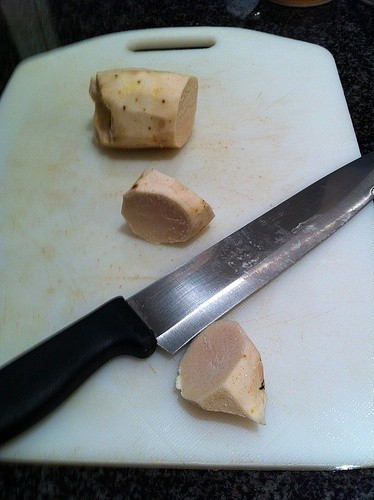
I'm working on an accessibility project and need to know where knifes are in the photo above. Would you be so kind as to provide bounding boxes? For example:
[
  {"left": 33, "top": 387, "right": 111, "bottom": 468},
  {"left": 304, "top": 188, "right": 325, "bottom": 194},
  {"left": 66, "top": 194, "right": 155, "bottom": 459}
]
[{"left": 0, "top": 155, "right": 374, "bottom": 446}]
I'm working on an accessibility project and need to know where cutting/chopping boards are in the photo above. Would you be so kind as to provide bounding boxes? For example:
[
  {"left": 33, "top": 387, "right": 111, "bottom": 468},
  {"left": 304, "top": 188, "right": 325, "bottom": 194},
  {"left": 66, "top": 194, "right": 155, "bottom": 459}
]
[{"left": 0, "top": 26, "right": 374, "bottom": 467}]
